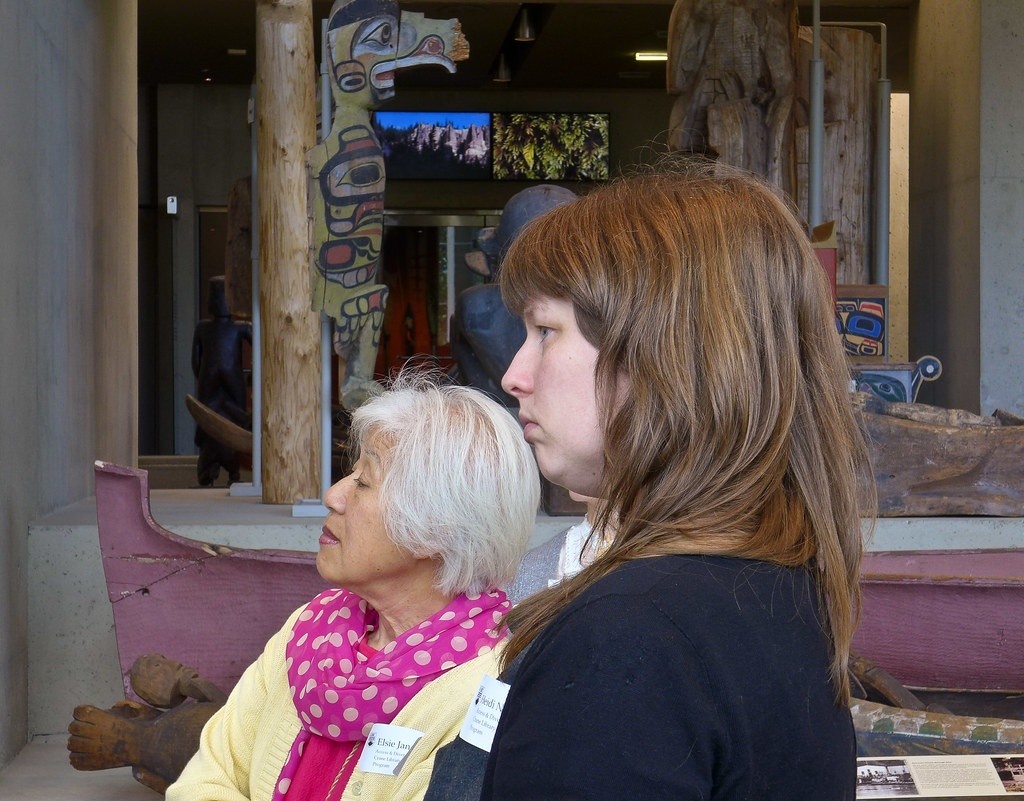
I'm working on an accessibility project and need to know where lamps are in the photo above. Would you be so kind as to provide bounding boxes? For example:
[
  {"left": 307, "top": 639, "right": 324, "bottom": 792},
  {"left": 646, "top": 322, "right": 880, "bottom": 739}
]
[
  {"left": 516, "top": 7, "right": 538, "bottom": 41},
  {"left": 494, "top": 52, "right": 510, "bottom": 81}
]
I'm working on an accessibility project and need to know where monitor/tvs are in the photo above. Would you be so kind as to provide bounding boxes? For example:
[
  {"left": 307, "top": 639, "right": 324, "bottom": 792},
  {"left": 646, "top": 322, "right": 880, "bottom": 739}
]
[{"left": 371, "top": 109, "right": 614, "bottom": 182}]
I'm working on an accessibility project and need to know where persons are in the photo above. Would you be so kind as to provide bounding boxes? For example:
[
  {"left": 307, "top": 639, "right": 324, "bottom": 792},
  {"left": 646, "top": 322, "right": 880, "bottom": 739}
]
[
  {"left": 162, "top": 384, "right": 543, "bottom": 801},
  {"left": 191, "top": 276, "right": 252, "bottom": 485},
  {"left": 450, "top": 186, "right": 580, "bottom": 408},
  {"left": 502, "top": 487, "right": 620, "bottom": 611},
  {"left": 421, "top": 168, "right": 882, "bottom": 801}
]
